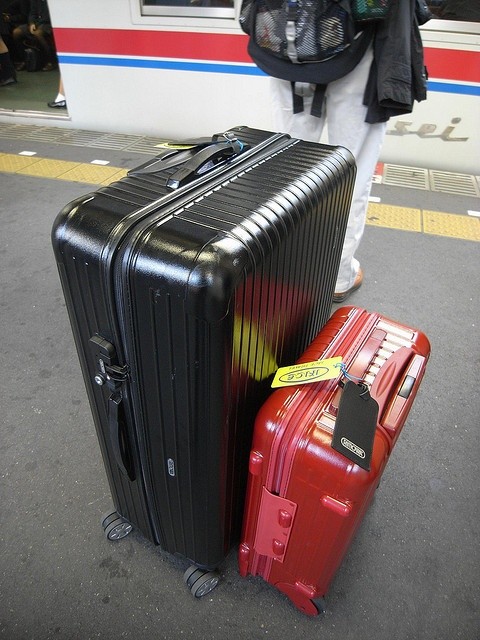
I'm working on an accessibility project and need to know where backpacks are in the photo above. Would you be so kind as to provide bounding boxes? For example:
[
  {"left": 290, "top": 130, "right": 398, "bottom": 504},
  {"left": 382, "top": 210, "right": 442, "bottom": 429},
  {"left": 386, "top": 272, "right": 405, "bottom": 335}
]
[{"left": 240, "top": 0, "right": 387, "bottom": 115}]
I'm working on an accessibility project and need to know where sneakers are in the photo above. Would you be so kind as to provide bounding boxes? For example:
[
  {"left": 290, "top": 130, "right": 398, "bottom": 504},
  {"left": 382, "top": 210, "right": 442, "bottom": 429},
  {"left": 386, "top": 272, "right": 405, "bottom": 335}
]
[{"left": 334, "top": 262, "right": 364, "bottom": 303}]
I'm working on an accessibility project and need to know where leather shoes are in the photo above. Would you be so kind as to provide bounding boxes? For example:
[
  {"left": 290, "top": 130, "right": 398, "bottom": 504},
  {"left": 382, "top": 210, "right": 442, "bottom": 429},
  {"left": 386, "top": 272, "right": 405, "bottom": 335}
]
[{"left": 48, "top": 100, "right": 66, "bottom": 109}]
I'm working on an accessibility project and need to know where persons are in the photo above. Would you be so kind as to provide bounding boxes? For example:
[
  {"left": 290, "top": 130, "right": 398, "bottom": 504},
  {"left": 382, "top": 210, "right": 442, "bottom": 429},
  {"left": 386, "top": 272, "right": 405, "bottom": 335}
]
[
  {"left": 48, "top": 76, "right": 67, "bottom": 108},
  {"left": 266, "top": 0, "right": 389, "bottom": 303},
  {"left": 12, "top": 0, "right": 56, "bottom": 71}
]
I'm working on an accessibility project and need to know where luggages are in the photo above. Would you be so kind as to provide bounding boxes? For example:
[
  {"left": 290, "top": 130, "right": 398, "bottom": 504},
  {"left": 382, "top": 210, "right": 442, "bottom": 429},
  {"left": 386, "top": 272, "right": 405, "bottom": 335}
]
[
  {"left": 237, "top": 307, "right": 430, "bottom": 613},
  {"left": 50, "top": 126, "right": 375, "bottom": 596}
]
